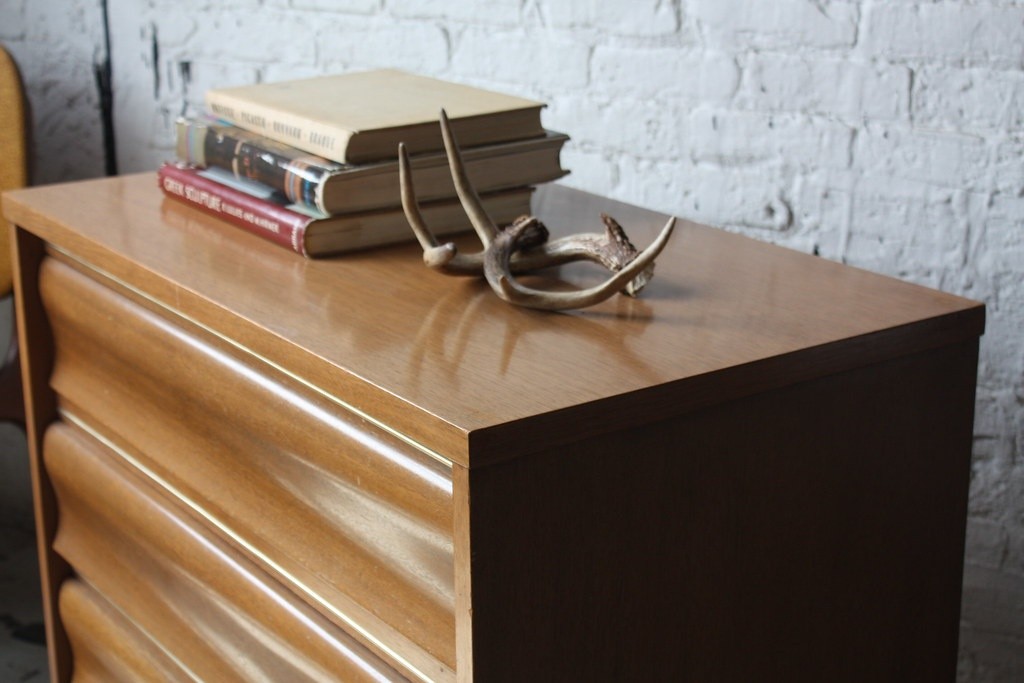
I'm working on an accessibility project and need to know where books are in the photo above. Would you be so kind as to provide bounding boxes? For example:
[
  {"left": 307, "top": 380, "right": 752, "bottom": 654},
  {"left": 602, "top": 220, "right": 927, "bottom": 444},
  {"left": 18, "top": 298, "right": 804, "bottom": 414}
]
[
  {"left": 205, "top": 65, "right": 546, "bottom": 165},
  {"left": 176, "top": 116, "right": 573, "bottom": 215},
  {"left": 157, "top": 162, "right": 538, "bottom": 260}
]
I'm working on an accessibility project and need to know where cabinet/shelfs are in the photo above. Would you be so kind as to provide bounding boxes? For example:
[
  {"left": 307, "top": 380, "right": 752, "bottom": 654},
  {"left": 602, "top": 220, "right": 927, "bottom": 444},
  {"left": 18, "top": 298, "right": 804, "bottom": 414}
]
[{"left": 0, "top": 168, "right": 986, "bottom": 683}]
